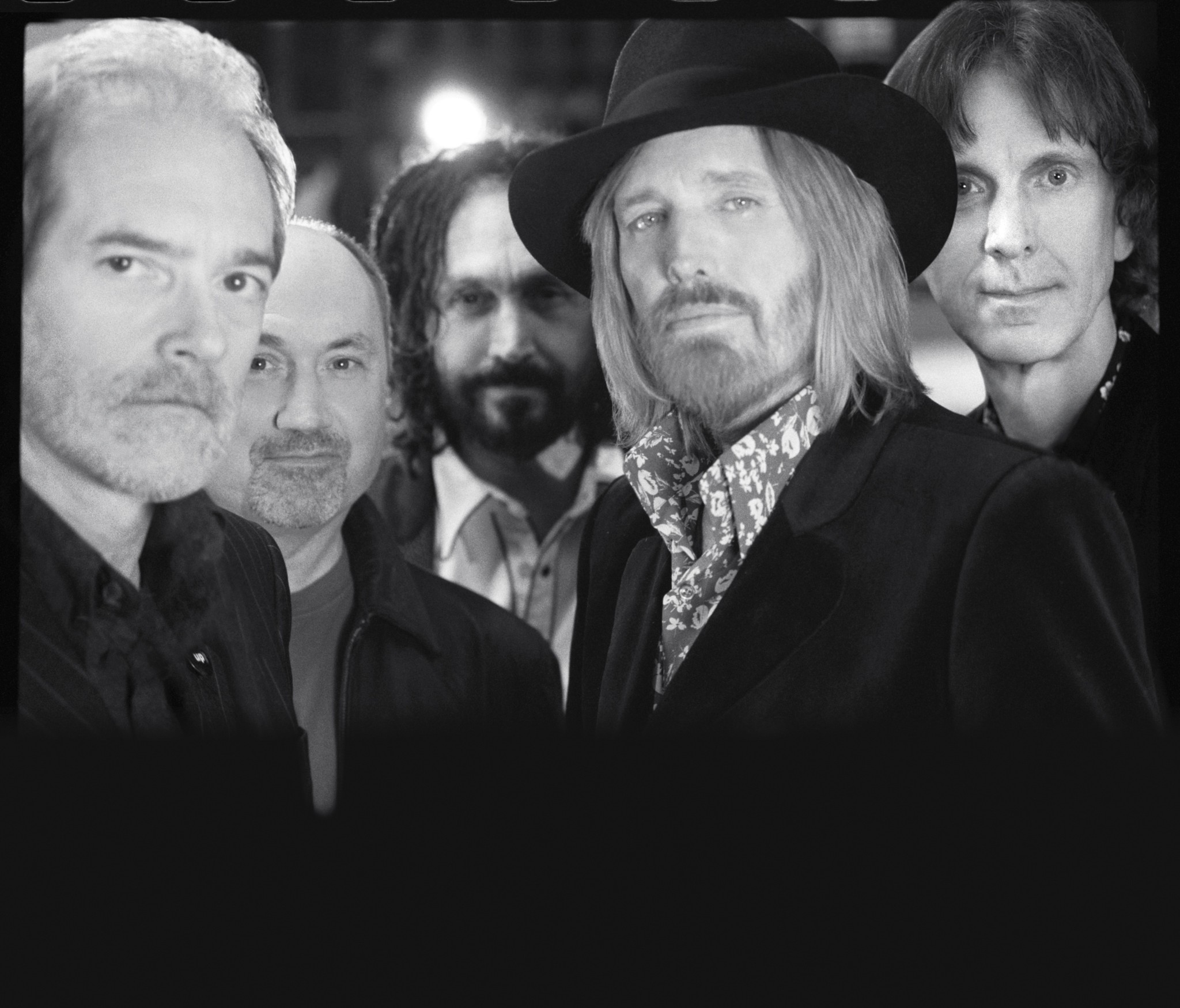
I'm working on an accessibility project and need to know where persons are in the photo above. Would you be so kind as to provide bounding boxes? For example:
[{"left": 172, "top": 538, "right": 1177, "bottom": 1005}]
[
  {"left": 878, "top": 0, "right": 1160, "bottom": 745},
  {"left": 203, "top": 219, "right": 575, "bottom": 1008},
  {"left": 21, "top": 19, "right": 341, "bottom": 1008},
  {"left": 508, "top": 18, "right": 1159, "bottom": 1008},
  {"left": 366, "top": 133, "right": 659, "bottom": 726}
]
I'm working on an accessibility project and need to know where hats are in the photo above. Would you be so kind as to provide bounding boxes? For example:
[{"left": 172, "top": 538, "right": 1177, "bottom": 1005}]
[{"left": 509, "top": 18, "right": 959, "bottom": 296}]
